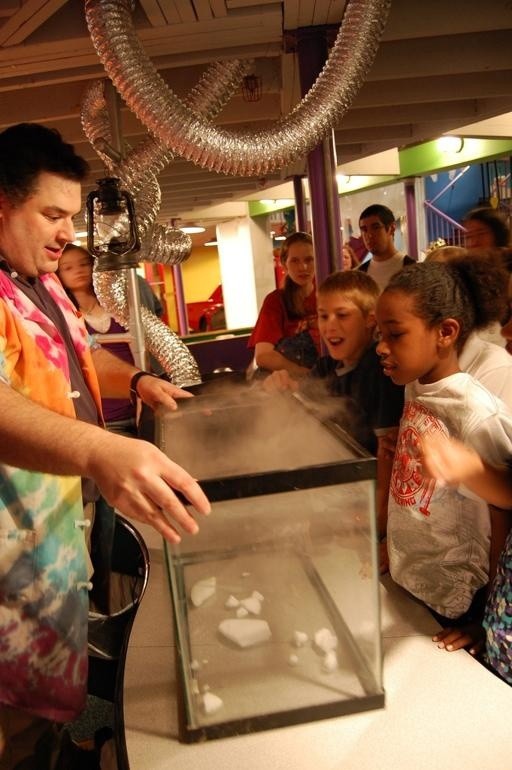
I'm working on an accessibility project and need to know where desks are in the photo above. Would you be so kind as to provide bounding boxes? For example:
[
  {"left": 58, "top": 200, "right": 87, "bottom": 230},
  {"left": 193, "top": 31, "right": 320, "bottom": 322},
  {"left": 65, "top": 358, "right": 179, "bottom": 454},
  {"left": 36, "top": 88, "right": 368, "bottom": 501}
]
[{"left": 114, "top": 504, "right": 512, "bottom": 765}]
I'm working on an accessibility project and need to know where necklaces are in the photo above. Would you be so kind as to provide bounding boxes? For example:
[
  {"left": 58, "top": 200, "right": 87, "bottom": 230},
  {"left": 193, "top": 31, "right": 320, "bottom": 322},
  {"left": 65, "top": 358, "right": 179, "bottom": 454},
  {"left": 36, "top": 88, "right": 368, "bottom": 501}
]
[{"left": 78, "top": 300, "right": 98, "bottom": 315}]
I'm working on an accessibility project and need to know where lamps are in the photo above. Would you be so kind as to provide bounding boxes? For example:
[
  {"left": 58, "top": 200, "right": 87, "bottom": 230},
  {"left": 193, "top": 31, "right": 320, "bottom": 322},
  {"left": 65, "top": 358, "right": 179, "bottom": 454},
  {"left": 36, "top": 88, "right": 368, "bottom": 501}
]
[{"left": 86, "top": 176, "right": 142, "bottom": 273}]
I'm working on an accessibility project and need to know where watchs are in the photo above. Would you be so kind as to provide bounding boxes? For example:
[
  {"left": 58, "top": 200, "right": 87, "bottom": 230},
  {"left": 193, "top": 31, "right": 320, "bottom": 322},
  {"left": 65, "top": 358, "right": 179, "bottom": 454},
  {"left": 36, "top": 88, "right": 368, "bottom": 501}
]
[{"left": 377, "top": 536, "right": 388, "bottom": 545}]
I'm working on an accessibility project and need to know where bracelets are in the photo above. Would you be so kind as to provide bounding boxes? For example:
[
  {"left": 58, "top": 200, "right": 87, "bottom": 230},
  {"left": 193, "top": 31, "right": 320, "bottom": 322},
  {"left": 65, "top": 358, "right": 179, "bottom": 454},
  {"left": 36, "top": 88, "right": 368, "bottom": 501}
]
[{"left": 129, "top": 370, "right": 160, "bottom": 406}]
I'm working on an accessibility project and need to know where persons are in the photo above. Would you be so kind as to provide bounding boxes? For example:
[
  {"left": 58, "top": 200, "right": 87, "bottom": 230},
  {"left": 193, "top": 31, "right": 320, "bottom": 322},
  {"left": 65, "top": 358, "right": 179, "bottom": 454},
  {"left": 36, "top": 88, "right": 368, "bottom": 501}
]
[
  {"left": 135, "top": 274, "right": 162, "bottom": 372},
  {"left": 0, "top": 122, "right": 211, "bottom": 769},
  {"left": 374, "top": 252, "right": 512, "bottom": 623},
  {"left": 343, "top": 204, "right": 418, "bottom": 291},
  {"left": 245, "top": 232, "right": 322, "bottom": 379},
  {"left": 55, "top": 243, "right": 156, "bottom": 440},
  {"left": 465, "top": 206, "right": 512, "bottom": 270},
  {"left": 341, "top": 244, "right": 361, "bottom": 271},
  {"left": 424, "top": 246, "right": 511, "bottom": 684},
  {"left": 312, "top": 270, "right": 404, "bottom": 576}
]
